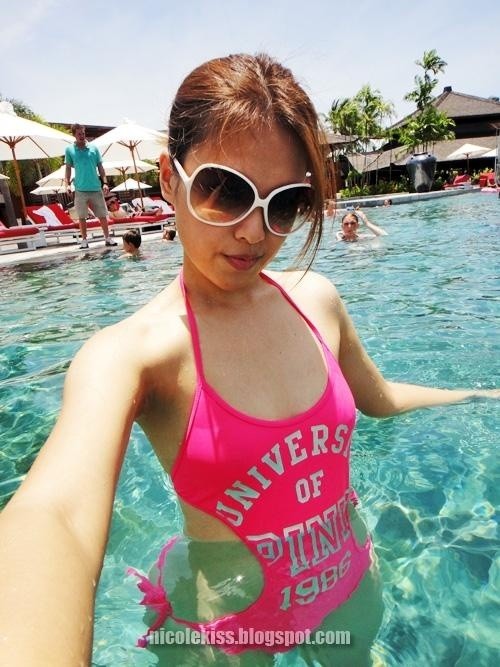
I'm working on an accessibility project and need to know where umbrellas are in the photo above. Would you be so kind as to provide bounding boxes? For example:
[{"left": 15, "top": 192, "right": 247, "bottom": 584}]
[
  {"left": 1, "top": 113, "right": 171, "bottom": 226},
  {"left": 447, "top": 143, "right": 492, "bottom": 175},
  {"left": 483, "top": 148, "right": 499, "bottom": 157}
]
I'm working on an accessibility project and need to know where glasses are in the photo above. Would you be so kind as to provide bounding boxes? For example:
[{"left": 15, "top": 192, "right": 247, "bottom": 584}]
[{"left": 173, "top": 156, "right": 317, "bottom": 238}]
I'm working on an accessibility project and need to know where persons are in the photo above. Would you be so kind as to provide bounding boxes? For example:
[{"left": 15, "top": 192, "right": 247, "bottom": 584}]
[
  {"left": 65, "top": 122, "right": 119, "bottom": 249},
  {"left": 383, "top": 198, "right": 393, "bottom": 208},
  {"left": 155, "top": 228, "right": 177, "bottom": 243},
  {"left": 325, "top": 200, "right": 339, "bottom": 216},
  {"left": 0, "top": 47, "right": 500, "bottom": 666},
  {"left": 336, "top": 209, "right": 388, "bottom": 243},
  {"left": 116, "top": 229, "right": 143, "bottom": 259}
]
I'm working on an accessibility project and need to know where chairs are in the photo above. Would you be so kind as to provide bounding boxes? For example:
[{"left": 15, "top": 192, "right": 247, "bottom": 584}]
[{"left": 0, "top": 195, "right": 176, "bottom": 255}]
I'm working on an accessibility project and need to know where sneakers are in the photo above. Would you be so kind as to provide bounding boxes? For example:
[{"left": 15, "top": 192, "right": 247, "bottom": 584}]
[
  {"left": 105, "top": 238, "right": 118, "bottom": 246},
  {"left": 80, "top": 239, "right": 89, "bottom": 249}
]
